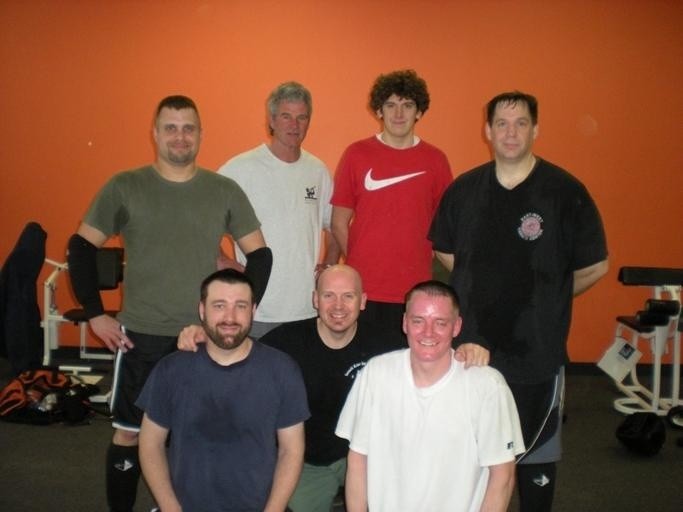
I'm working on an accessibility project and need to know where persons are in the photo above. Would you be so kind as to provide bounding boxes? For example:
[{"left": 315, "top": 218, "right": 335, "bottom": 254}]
[
  {"left": 134, "top": 270, "right": 313, "bottom": 512},
  {"left": 334, "top": 280, "right": 526, "bottom": 512},
  {"left": 427, "top": 90, "right": 611, "bottom": 512},
  {"left": 65, "top": 94, "right": 273, "bottom": 511},
  {"left": 177, "top": 264, "right": 492, "bottom": 512},
  {"left": 328, "top": 67, "right": 454, "bottom": 357},
  {"left": 215, "top": 81, "right": 336, "bottom": 341}
]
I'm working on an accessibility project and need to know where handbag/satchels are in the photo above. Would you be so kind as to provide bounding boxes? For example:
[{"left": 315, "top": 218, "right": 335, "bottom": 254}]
[{"left": 0, "top": 365, "right": 100, "bottom": 425}]
